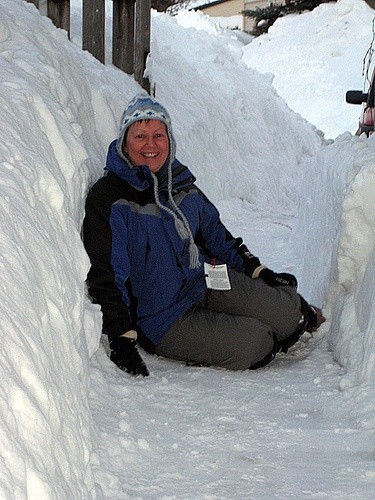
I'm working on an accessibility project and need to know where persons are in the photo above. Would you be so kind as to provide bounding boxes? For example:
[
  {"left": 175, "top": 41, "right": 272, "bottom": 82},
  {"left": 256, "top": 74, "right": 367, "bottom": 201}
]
[{"left": 80, "top": 92, "right": 325, "bottom": 379}]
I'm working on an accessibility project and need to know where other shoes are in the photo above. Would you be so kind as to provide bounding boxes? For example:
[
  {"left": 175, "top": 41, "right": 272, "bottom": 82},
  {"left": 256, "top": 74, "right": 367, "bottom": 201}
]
[{"left": 305, "top": 303, "right": 326, "bottom": 333}]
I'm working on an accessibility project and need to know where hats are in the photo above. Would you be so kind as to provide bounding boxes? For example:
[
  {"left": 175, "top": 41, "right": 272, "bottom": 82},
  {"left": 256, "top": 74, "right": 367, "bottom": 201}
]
[{"left": 117, "top": 92, "right": 202, "bottom": 269}]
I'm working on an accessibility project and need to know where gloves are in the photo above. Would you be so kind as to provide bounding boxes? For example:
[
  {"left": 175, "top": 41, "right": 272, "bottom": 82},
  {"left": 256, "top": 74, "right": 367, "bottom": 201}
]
[
  {"left": 259, "top": 267, "right": 297, "bottom": 288},
  {"left": 110, "top": 337, "right": 149, "bottom": 376}
]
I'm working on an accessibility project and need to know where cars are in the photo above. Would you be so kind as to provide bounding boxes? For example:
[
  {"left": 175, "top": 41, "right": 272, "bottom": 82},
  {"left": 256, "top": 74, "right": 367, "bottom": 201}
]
[{"left": 346, "top": 67, "right": 375, "bottom": 137}]
